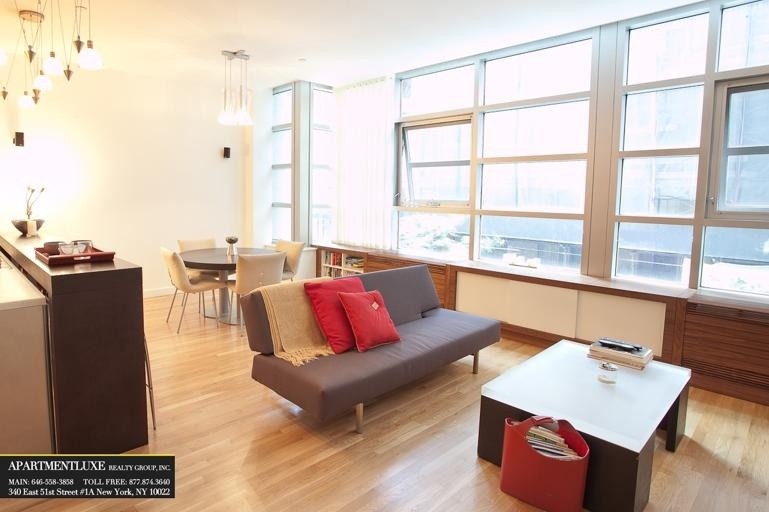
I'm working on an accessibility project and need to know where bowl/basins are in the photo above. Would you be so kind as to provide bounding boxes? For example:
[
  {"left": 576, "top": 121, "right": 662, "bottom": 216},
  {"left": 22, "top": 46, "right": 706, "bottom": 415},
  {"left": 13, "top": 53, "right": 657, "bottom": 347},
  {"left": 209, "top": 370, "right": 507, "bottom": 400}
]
[{"left": 44, "top": 242, "right": 64, "bottom": 255}]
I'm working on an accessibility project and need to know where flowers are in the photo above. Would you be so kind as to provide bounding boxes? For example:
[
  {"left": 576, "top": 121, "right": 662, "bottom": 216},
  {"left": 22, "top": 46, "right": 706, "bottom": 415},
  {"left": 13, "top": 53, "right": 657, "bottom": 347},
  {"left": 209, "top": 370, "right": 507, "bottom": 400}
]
[{"left": 24, "top": 185, "right": 46, "bottom": 219}]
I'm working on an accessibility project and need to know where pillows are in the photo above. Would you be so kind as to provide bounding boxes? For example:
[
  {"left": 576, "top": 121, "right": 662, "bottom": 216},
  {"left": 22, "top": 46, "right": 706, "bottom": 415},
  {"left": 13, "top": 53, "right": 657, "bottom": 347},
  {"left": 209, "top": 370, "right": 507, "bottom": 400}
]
[
  {"left": 337, "top": 289, "right": 401, "bottom": 353},
  {"left": 303, "top": 277, "right": 366, "bottom": 355}
]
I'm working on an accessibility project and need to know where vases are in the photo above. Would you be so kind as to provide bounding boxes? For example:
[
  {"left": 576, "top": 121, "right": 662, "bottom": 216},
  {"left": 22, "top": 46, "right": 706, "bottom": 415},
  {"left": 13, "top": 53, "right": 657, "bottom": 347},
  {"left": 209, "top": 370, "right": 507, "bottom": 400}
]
[{"left": 11, "top": 219, "right": 45, "bottom": 236}]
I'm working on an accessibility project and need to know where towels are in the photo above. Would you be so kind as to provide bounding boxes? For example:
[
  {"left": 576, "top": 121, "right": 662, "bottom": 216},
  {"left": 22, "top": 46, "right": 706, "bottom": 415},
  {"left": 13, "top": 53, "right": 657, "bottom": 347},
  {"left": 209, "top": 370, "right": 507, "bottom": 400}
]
[{"left": 26, "top": 220, "right": 38, "bottom": 238}]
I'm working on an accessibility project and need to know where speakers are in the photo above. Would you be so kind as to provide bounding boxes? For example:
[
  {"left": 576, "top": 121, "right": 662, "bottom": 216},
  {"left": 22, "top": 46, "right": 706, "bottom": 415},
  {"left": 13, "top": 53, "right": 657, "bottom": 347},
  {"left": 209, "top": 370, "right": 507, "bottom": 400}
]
[
  {"left": 14, "top": 131, "right": 25, "bottom": 147},
  {"left": 223, "top": 146, "right": 231, "bottom": 157}
]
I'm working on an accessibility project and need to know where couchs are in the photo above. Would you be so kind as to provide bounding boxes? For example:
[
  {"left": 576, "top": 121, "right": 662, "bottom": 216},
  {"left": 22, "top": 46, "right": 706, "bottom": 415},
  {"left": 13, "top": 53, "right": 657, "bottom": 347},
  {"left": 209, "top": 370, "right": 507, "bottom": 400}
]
[{"left": 238, "top": 264, "right": 500, "bottom": 434}]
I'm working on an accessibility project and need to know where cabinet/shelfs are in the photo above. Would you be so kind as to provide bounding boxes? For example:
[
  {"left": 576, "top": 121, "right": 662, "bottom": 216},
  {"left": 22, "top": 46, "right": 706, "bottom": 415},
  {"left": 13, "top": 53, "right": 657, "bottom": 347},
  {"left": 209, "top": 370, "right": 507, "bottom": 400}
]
[{"left": 320, "top": 249, "right": 365, "bottom": 279}]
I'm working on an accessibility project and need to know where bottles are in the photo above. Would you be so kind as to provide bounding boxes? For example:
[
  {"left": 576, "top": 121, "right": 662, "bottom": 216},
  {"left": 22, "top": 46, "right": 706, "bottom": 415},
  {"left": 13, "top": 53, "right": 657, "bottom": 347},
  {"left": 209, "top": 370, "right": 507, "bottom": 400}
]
[{"left": 598, "top": 362, "right": 618, "bottom": 384}]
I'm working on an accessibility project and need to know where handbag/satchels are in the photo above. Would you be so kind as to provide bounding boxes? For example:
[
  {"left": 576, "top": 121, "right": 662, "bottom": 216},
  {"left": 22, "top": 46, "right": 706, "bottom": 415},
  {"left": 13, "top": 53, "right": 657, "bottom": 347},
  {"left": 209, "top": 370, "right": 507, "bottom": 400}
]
[{"left": 500, "top": 414, "right": 590, "bottom": 512}]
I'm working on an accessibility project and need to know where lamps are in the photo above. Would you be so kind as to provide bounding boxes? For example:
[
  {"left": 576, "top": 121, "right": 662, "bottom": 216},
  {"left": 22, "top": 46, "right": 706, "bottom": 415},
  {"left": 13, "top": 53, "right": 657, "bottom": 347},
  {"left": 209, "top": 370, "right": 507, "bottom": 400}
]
[
  {"left": 219, "top": 50, "right": 254, "bottom": 127},
  {"left": 2, "top": 0, "right": 102, "bottom": 112}
]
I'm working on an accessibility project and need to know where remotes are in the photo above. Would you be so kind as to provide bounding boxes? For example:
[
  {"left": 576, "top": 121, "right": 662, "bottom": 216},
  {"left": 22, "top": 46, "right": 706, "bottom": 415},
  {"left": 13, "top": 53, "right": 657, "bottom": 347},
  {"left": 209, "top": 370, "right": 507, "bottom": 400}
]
[
  {"left": 598, "top": 340, "right": 634, "bottom": 351},
  {"left": 602, "top": 338, "right": 642, "bottom": 351}
]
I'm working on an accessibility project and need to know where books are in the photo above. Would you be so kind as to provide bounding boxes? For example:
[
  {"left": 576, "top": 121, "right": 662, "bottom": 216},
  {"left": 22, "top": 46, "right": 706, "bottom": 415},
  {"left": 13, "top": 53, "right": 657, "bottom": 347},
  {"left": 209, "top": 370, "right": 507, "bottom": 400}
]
[
  {"left": 325, "top": 251, "right": 365, "bottom": 278},
  {"left": 510, "top": 420, "right": 583, "bottom": 460},
  {"left": 587, "top": 337, "right": 654, "bottom": 371}
]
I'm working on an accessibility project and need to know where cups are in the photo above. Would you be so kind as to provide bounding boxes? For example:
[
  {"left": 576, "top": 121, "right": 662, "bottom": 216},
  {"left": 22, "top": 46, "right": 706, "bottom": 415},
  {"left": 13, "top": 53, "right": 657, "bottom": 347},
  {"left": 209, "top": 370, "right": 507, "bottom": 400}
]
[
  {"left": 511, "top": 253, "right": 540, "bottom": 267},
  {"left": 59, "top": 240, "right": 93, "bottom": 255},
  {"left": 27, "top": 218, "right": 37, "bottom": 237}
]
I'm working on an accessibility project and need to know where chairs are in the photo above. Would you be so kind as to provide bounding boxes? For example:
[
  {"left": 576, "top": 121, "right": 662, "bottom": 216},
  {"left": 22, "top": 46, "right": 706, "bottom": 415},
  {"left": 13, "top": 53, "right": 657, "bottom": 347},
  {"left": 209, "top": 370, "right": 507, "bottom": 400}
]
[
  {"left": 179, "top": 239, "right": 216, "bottom": 307},
  {"left": 226, "top": 252, "right": 287, "bottom": 337},
  {"left": 160, "top": 247, "right": 228, "bottom": 334},
  {"left": 275, "top": 238, "right": 304, "bottom": 282}
]
